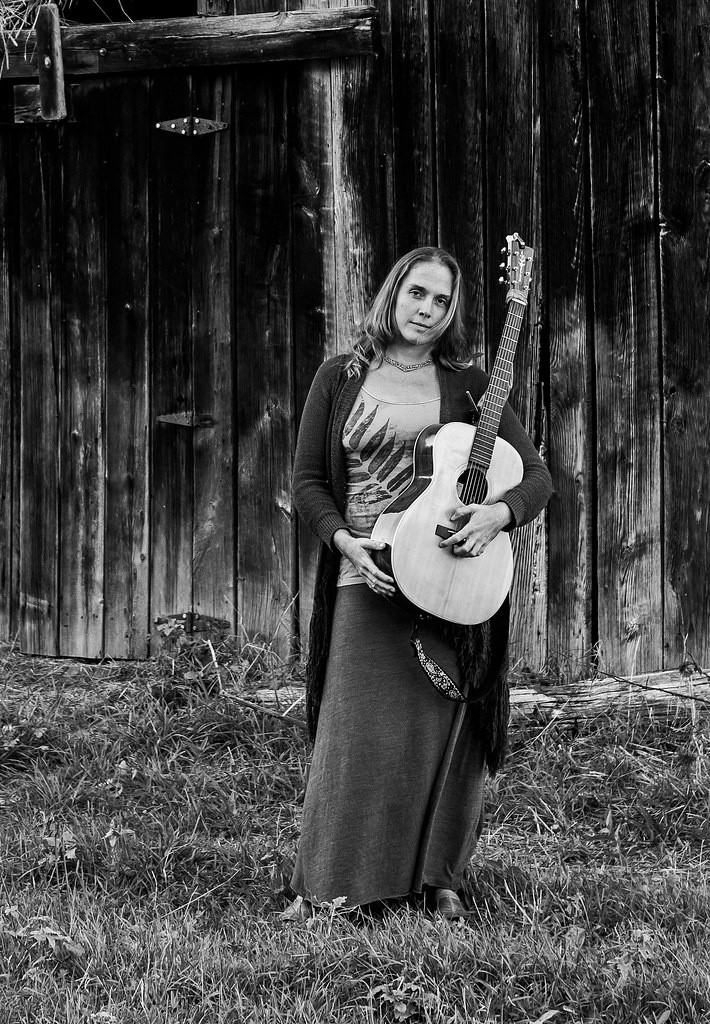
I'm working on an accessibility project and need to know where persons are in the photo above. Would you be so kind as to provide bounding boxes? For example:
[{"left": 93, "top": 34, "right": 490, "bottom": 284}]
[{"left": 275, "top": 245, "right": 552, "bottom": 922}]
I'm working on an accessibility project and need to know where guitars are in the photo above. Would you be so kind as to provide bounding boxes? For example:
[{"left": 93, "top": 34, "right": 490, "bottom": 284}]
[{"left": 370, "top": 232, "right": 535, "bottom": 628}]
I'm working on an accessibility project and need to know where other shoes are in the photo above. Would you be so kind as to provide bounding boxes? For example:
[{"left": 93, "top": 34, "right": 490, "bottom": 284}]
[
  {"left": 434, "top": 890, "right": 468, "bottom": 921},
  {"left": 278, "top": 898, "right": 312, "bottom": 922}
]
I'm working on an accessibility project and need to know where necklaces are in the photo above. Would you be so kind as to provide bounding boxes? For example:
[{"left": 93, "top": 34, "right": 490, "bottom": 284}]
[{"left": 382, "top": 351, "right": 434, "bottom": 372}]
